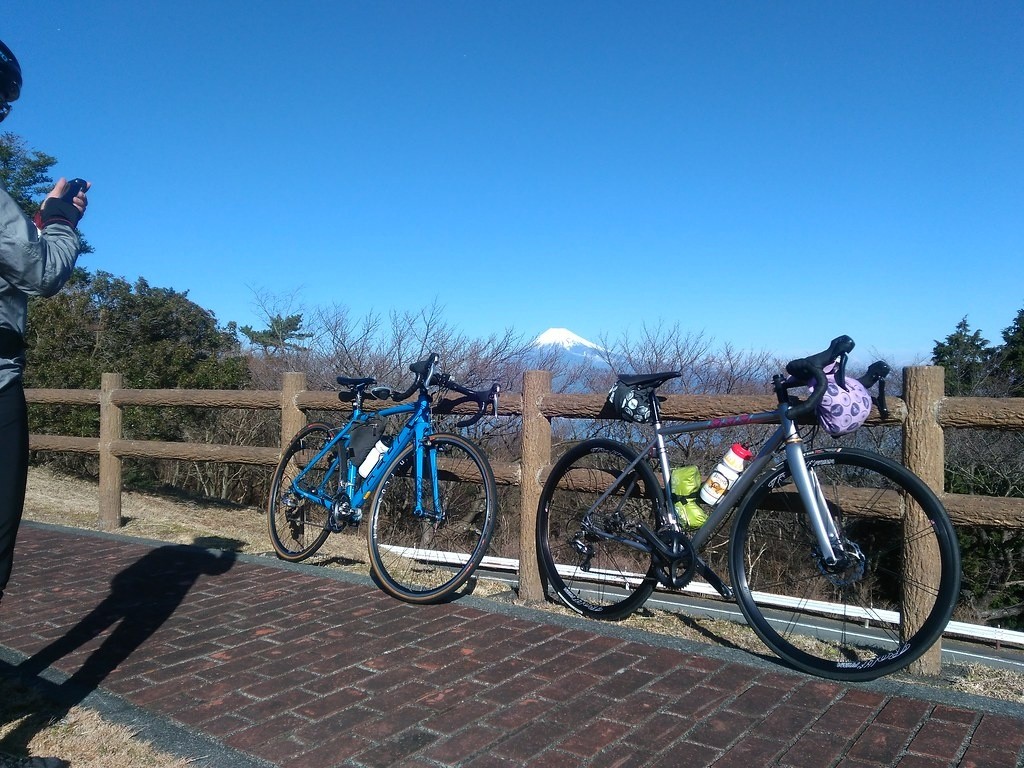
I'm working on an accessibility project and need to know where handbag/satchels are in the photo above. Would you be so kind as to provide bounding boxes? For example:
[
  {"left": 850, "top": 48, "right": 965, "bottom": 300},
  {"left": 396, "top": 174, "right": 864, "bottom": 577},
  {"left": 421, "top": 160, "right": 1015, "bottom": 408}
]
[{"left": 349, "top": 412, "right": 388, "bottom": 466}]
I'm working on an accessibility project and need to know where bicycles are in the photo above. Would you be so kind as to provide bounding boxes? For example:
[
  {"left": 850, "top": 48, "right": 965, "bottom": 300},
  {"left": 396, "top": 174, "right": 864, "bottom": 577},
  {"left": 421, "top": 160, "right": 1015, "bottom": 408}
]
[
  {"left": 534, "top": 334, "right": 964, "bottom": 683},
  {"left": 264, "top": 351, "right": 503, "bottom": 606}
]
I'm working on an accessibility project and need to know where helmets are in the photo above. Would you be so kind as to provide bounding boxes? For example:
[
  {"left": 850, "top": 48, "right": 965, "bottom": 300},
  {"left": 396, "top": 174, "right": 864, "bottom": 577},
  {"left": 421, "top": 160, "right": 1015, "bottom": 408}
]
[{"left": 0, "top": 40, "right": 22, "bottom": 122}]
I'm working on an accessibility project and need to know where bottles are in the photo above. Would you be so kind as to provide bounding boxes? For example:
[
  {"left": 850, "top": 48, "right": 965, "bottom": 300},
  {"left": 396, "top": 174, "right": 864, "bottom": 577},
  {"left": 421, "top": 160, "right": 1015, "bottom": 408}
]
[
  {"left": 700, "top": 442, "right": 752, "bottom": 507},
  {"left": 358, "top": 436, "right": 393, "bottom": 479}
]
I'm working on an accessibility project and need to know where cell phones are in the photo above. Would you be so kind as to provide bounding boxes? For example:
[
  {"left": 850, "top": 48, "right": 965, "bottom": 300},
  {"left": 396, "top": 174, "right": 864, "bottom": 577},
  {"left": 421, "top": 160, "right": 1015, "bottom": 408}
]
[{"left": 60, "top": 177, "right": 87, "bottom": 205}]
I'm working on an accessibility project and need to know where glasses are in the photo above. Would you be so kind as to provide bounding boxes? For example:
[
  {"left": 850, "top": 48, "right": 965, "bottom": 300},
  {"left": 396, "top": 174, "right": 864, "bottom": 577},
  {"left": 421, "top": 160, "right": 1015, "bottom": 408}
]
[{"left": 0, "top": 93, "right": 12, "bottom": 122}]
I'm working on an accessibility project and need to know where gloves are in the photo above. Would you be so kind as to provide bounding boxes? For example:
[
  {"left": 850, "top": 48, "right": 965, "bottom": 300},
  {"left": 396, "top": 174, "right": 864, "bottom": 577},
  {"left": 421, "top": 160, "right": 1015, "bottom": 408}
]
[{"left": 34, "top": 198, "right": 80, "bottom": 231}]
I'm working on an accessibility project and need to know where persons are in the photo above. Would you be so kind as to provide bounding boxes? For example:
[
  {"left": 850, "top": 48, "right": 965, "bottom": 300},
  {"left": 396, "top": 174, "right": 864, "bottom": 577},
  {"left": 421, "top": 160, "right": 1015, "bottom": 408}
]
[{"left": 0, "top": 41, "right": 90, "bottom": 600}]
